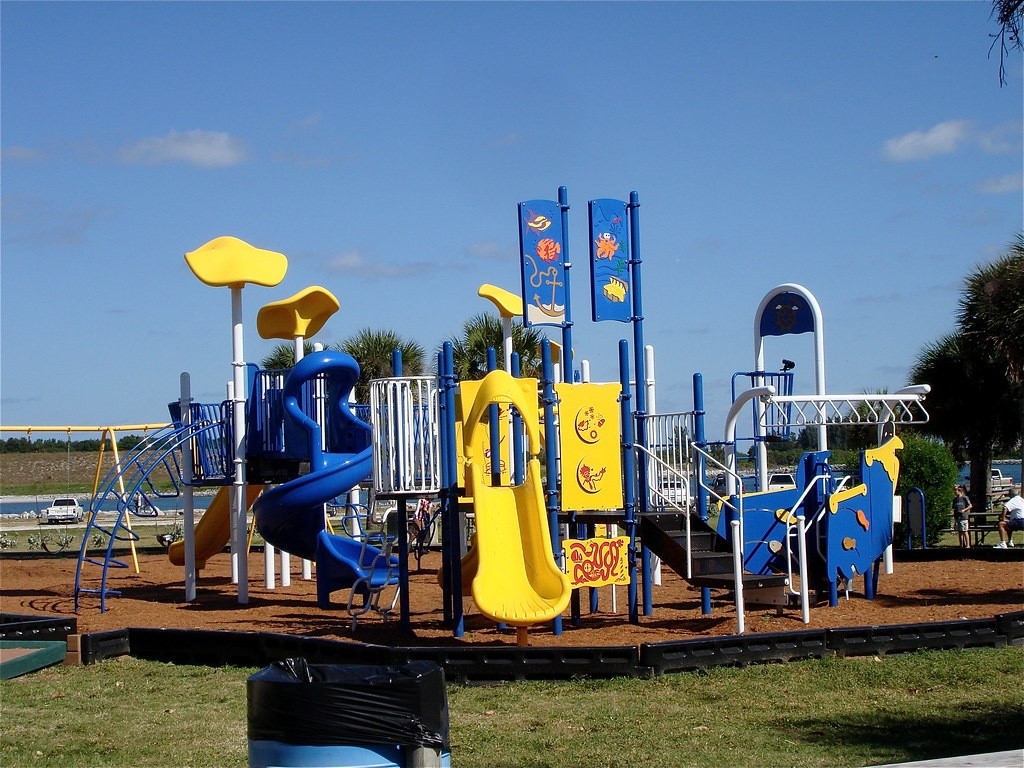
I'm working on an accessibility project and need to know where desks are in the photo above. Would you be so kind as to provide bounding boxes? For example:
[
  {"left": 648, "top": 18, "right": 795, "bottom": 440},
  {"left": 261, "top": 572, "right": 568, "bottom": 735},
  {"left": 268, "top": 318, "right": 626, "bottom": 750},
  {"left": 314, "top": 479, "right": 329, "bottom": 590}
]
[{"left": 940, "top": 513, "right": 1011, "bottom": 546}]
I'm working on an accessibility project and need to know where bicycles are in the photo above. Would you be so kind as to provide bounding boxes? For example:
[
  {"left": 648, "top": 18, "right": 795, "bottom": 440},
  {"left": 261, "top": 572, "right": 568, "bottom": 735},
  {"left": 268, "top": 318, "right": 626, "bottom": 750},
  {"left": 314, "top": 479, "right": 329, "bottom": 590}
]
[{"left": 127, "top": 499, "right": 154, "bottom": 515}]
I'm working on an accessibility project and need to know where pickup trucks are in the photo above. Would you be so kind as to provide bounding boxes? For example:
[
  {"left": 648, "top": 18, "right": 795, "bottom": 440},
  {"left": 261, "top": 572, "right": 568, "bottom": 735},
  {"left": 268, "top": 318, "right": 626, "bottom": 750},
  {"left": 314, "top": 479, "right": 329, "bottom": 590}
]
[
  {"left": 964, "top": 468, "right": 1013, "bottom": 492},
  {"left": 46, "top": 497, "right": 84, "bottom": 524}
]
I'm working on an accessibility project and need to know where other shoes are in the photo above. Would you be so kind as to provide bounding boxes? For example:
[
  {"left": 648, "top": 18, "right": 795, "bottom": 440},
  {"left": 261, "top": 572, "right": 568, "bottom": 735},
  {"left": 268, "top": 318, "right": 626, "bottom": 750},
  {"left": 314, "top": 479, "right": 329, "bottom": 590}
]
[{"left": 966, "top": 546, "right": 970, "bottom": 548}]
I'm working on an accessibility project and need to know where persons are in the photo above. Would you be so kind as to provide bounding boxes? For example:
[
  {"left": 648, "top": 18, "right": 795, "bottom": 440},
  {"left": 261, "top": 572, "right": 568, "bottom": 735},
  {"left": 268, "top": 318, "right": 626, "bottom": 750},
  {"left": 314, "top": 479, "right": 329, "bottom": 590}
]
[
  {"left": 993, "top": 488, "right": 1024, "bottom": 549},
  {"left": 951, "top": 485, "right": 973, "bottom": 549}
]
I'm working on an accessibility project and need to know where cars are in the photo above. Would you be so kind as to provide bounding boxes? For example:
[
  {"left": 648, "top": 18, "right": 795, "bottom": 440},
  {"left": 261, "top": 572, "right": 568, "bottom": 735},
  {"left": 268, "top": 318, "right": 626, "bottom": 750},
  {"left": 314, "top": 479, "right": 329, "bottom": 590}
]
[
  {"left": 767, "top": 472, "right": 797, "bottom": 491},
  {"left": 658, "top": 480, "right": 686, "bottom": 505},
  {"left": 833, "top": 476, "right": 848, "bottom": 491},
  {"left": 708, "top": 472, "right": 746, "bottom": 504}
]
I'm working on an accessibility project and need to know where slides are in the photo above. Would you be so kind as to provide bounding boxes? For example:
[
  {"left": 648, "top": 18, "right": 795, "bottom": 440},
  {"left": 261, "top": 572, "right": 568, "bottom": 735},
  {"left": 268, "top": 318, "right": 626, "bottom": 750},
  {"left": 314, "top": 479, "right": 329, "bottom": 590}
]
[
  {"left": 438, "top": 369, "right": 573, "bottom": 627},
  {"left": 251, "top": 350, "right": 410, "bottom": 602},
  {"left": 168, "top": 440, "right": 262, "bottom": 567}
]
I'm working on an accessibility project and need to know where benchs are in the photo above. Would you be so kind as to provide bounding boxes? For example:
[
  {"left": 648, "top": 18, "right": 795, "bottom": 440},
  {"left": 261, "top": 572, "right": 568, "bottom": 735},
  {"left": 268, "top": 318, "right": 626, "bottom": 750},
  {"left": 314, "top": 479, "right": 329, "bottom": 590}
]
[
  {"left": 941, "top": 529, "right": 984, "bottom": 546},
  {"left": 970, "top": 526, "right": 1008, "bottom": 546}
]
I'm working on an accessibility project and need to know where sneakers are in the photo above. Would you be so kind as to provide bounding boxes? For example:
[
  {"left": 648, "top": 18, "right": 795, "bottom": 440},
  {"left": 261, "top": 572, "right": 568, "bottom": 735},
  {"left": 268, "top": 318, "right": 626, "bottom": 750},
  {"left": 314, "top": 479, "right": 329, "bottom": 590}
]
[
  {"left": 993, "top": 543, "right": 1007, "bottom": 549},
  {"left": 1006, "top": 542, "right": 1014, "bottom": 547}
]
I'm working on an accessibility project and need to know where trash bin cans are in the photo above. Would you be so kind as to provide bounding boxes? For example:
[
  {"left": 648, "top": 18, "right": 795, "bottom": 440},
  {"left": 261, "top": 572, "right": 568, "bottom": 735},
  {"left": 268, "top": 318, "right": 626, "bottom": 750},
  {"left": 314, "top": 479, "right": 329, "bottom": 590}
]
[{"left": 246, "top": 663, "right": 454, "bottom": 768}]
